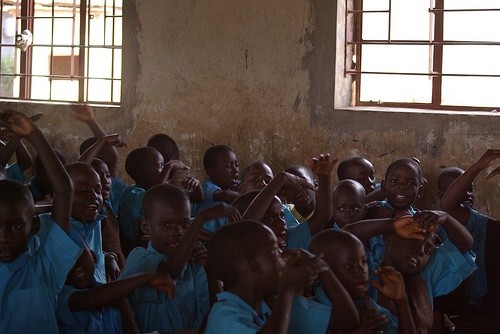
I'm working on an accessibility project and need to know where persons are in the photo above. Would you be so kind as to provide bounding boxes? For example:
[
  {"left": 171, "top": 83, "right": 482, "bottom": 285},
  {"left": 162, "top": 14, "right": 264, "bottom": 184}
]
[
  {"left": 202, "top": 219, "right": 360, "bottom": 334},
  {"left": 0, "top": 102, "right": 500, "bottom": 334}
]
